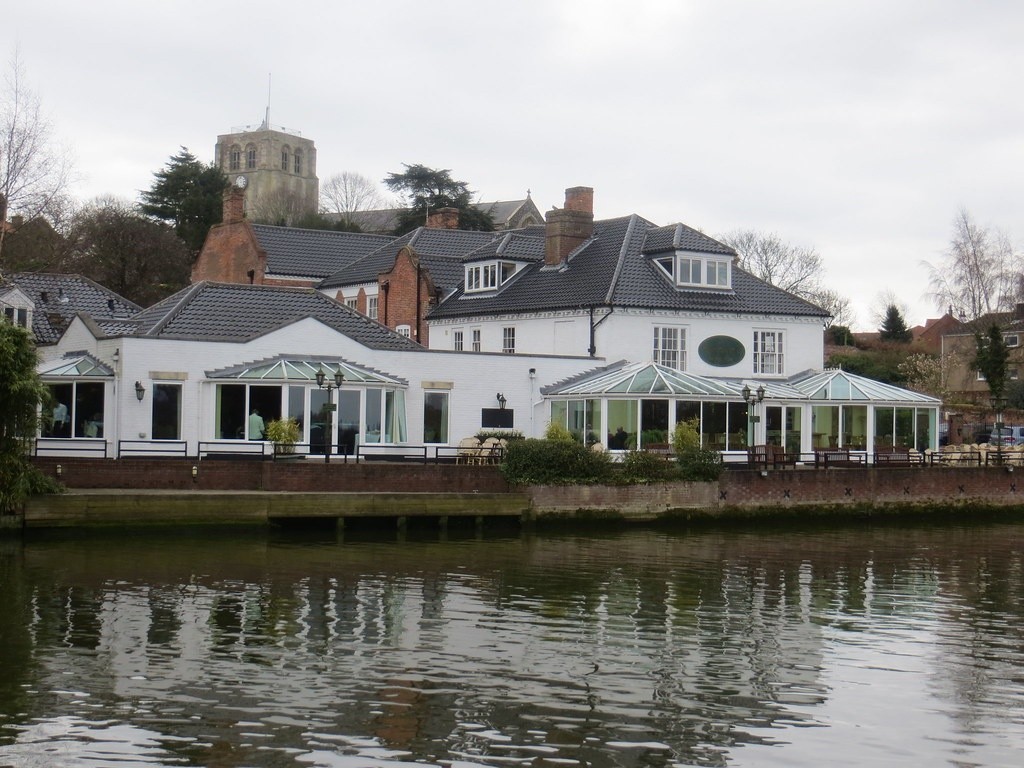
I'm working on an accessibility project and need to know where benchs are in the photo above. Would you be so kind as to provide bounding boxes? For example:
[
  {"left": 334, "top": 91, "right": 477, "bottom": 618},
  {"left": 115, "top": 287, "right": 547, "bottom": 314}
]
[
  {"left": 747, "top": 444, "right": 796, "bottom": 470},
  {"left": 815, "top": 447, "right": 862, "bottom": 467},
  {"left": 876, "top": 446, "right": 922, "bottom": 467}
]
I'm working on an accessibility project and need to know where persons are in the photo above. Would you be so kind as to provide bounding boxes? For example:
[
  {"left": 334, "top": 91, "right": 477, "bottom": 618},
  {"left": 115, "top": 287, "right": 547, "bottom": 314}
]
[
  {"left": 249, "top": 409, "right": 266, "bottom": 441},
  {"left": 51, "top": 398, "right": 67, "bottom": 438}
]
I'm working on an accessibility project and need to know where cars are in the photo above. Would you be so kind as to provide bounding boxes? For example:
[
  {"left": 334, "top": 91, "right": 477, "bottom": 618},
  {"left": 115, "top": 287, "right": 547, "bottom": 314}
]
[{"left": 989, "top": 426, "right": 1024, "bottom": 446}]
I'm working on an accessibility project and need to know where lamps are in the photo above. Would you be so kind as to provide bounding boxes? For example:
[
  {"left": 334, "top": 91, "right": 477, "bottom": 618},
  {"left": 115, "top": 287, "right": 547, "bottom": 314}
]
[
  {"left": 192, "top": 466, "right": 198, "bottom": 484},
  {"left": 135, "top": 381, "right": 145, "bottom": 402},
  {"left": 56, "top": 464, "right": 62, "bottom": 477},
  {"left": 497, "top": 392, "right": 506, "bottom": 412}
]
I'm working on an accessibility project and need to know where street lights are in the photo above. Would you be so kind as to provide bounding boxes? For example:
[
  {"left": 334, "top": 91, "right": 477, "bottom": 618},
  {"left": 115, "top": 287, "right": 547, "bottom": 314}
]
[
  {"left": 742, "top": 384, "right": 765, "bottom": 446},
  {"left": 989, "top": 393, "right": 1009, "bottom": 464}
]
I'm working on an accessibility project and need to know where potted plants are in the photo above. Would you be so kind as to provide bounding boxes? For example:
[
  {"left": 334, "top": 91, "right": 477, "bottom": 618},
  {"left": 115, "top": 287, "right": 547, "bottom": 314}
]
[{"left": 267, "top": 416, "right": 302, "bottom": 462}]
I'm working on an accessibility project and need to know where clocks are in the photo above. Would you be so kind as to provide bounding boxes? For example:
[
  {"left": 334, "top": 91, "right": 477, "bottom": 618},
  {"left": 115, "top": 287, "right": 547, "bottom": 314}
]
[{"left": 235, "top": 175, "right": 248, "bottom": 190}]
